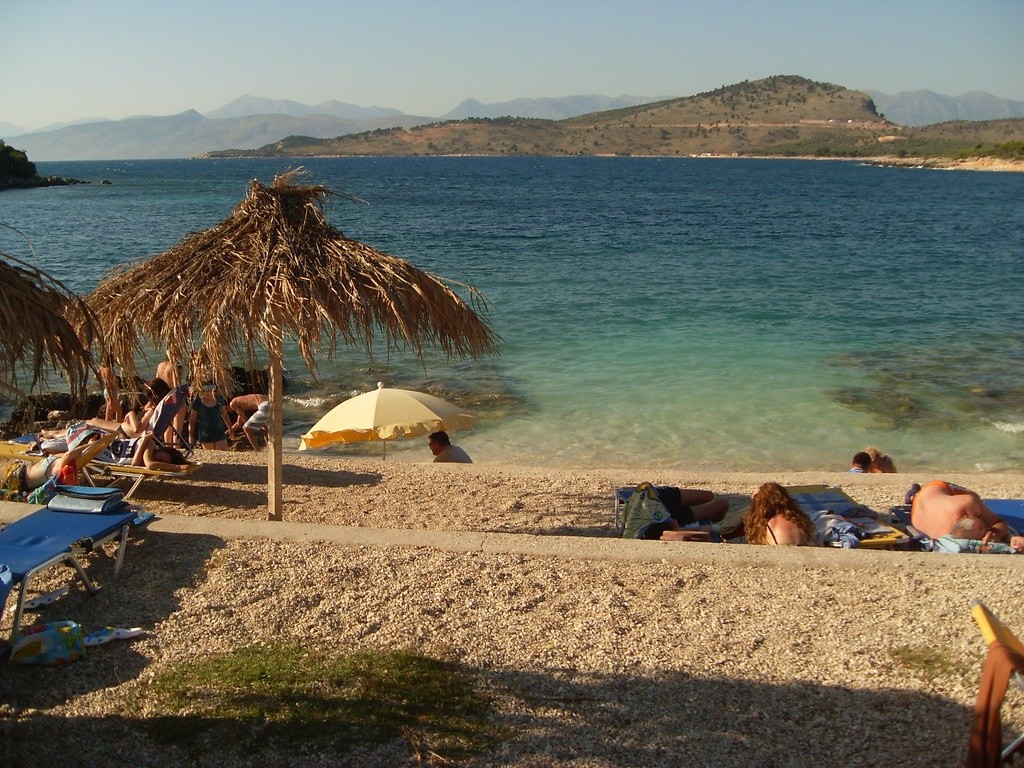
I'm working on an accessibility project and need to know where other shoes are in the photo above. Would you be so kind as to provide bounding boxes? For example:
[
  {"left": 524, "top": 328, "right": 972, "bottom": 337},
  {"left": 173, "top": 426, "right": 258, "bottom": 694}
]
[{"left": 904, "top": 483, "right": 921, "bottom": 504}]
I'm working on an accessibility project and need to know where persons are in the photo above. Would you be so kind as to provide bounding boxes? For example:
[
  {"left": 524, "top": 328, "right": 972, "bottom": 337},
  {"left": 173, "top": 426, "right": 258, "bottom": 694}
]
[
  {"left": 0, "top": 378, "right": 185, "bottom": 492},
  {"left": 155, "top": 348, "right": 185, "bottom": 449},
  {"left": 904, "top": 480, "right": 1024, "bottom": 555},
  {"left": 428, "top": 430, "right": 473, "bottom": 463},
  {"left": 622, "top": 482, "right": 729, "bottom": 539},
  {"left": 848, "top": 447, "right": 897, "bottom": 473},
  {"left": 224, "top": 394, "right": 269, "bottom": 452},
  {"left": 743, "top": 481, "right": 816, "bottom": 546},
  {"left": 188, "top": 381, "right": 234, "bottom": 450},
  {"left": 93, "top": 352, "right": 122, "bottom": 422}
]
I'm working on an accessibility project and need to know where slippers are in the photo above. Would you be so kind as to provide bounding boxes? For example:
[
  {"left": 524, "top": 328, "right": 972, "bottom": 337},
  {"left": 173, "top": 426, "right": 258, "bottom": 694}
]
[
  {"left": 83, "top": 626, "right": 142, "bottom": 647},
  {"left": 23, "top": 587, "right": 68, "bottom": 609}
]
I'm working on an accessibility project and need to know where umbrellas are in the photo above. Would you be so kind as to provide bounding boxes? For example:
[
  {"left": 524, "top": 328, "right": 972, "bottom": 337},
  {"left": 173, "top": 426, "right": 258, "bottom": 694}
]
[
  {"left": 298, "top": 382, "right": 479, "bottom": 461},
  {"left": 0, "top": 250, "right": 125, "bottom": 437},
  {"left": 55, "top": 165, "right": 507, "bottom": 521}
]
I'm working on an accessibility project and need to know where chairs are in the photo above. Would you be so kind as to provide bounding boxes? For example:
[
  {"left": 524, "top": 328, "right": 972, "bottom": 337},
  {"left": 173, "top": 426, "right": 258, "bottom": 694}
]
[
  {"left": 887, "top": 505, "right": 1020, "bottom": 554},
  {"left": 0, "top": 383, "right": 195, "bottom": 645},
  {"left": 614, "top": 485, "right": 724, "bottom": 544},
  {"left": 787, "top": 485, "right": 911, "bottom": 552},
  {"left": 972, "top": 603, "right": 1024, "bottom": 768}
]
